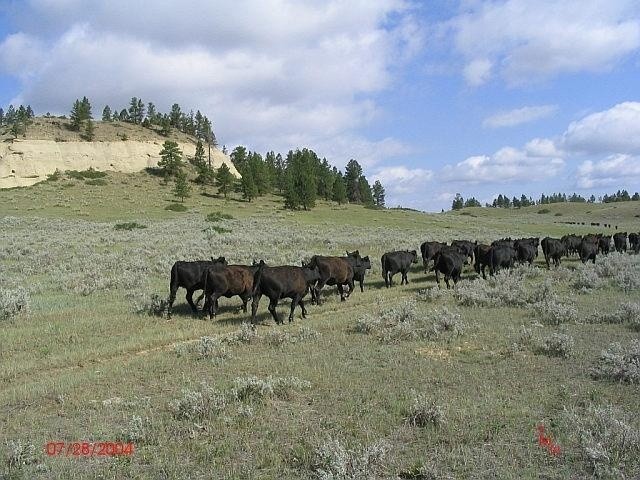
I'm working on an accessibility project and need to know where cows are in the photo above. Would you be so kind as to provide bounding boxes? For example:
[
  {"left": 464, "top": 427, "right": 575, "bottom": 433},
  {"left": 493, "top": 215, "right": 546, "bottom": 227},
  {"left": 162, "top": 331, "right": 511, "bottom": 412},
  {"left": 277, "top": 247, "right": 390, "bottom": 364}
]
[
  {"left": 166, "top": 256, "right": 229, "bottom": 316},
  {"left": 195, "top": 258, "right": 266, "bottom": 320},
  {"left": 381, "top": 249, "right": 418, "bottom": 287},
  {"left": 309, "top": 250, "right": 371, "bottom": 305},
  {"left": 421, "top": 231, "right": 640, "bottom": 288},
  {"left": 248, "top": 260, "right": 321, "bottom": 326}
]
